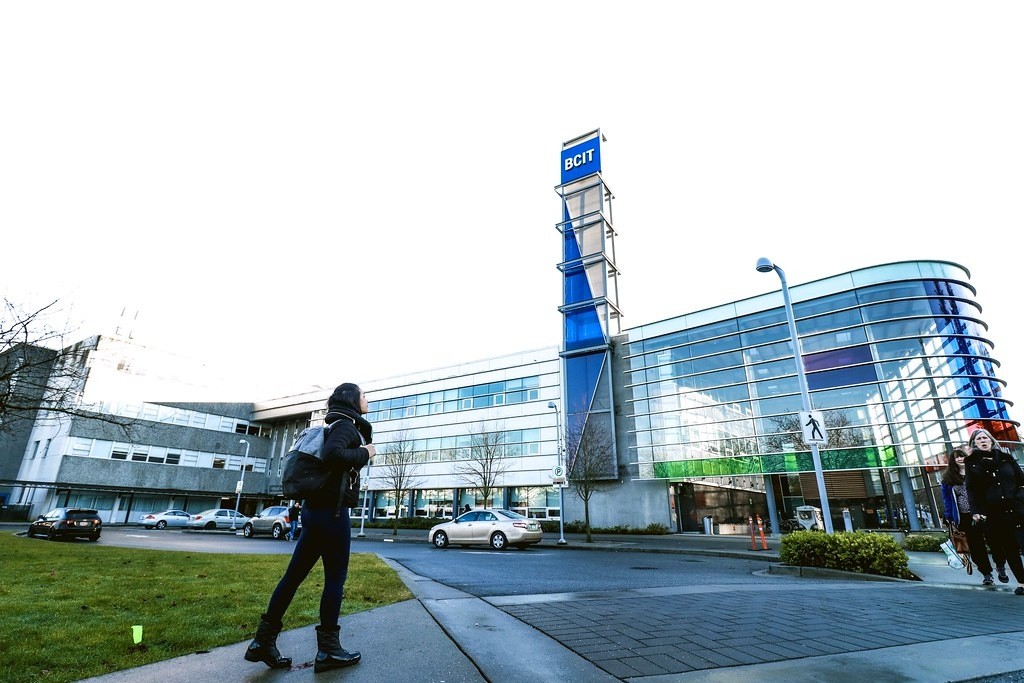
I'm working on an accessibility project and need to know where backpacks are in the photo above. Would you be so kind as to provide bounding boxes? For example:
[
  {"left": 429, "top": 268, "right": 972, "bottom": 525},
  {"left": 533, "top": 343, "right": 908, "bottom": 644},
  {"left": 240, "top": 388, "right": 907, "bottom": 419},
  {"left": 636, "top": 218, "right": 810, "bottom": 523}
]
[{"left": 282, "top": 419, "right": 366, "bottom": 500}]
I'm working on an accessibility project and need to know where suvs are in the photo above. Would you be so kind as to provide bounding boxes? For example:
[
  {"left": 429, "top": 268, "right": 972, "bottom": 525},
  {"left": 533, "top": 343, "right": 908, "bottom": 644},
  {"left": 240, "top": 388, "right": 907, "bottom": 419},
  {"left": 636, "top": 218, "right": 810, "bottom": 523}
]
[{"left": 242, "top": 506, "right": 303, "bottom": 540}]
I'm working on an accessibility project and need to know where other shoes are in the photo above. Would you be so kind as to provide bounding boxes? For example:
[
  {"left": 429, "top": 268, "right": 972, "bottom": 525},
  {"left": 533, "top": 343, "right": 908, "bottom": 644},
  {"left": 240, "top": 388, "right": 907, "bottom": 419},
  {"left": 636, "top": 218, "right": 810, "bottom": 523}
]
[
  {"left": 289, "top": 538, "right": 293, "bottom": 542},
  {"left": 995, "top": 565, "right": 1009, "bottom": 583},
  {"left": 285, "top": 534, "right": 288, "bottom": 540},
  {"left": 1014, "top": 586, "right": 1024, "bottom": 595},
  {"left": 983, "top": 574, "right": 995, "bottom": 586}
]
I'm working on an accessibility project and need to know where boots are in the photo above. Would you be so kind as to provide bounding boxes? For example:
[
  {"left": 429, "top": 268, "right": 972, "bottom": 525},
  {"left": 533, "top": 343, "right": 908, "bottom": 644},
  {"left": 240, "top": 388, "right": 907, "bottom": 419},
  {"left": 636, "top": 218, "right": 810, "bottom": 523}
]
[
  {"left": 244, "top": 614, "right": 292, "bottom": 669},
  {"left": 314, "top": 625, "right": 361, "bottom": 673}
]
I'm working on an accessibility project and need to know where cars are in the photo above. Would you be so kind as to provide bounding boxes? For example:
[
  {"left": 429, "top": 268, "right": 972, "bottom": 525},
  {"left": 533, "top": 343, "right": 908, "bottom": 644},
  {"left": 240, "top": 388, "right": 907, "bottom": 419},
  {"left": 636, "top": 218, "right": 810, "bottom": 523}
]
[
  {"left": 428, "top": 509, "right": 543, "bottom": 550},
  {"left": 27, "top": 507, "right": 103, "bottom": 542},
  {"left": 137, "top": 509, "right": 192, "bottom": 530},
  {"left": 185, "top": 508, "right": 251, "bottom": 531}
]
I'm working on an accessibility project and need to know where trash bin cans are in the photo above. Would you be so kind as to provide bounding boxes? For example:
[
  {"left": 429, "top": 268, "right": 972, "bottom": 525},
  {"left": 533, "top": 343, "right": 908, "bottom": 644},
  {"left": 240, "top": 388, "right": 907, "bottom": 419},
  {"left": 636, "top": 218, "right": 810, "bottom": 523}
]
[{"left": 702, "top": 515, "right": 713, "bottom": 534}]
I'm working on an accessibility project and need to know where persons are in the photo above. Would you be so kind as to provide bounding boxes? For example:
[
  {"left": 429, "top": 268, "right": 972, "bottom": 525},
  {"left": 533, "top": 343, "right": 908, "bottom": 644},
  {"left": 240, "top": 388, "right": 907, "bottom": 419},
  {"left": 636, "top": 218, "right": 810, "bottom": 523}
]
[
  {"left": 285, "top": 501, "right": 302, "bottom": 541},
  {"left": 940, "top": 449, "right": 1009, "bottom": 585},
  {"left": 462, "top": 504, "right": 471, "bottom": 514},
  {"left": 963, "top": 428, "right": 1024, "bottom": 595},
  {"left": 244, "top": 383, "right": 376, "bottom": 673}
]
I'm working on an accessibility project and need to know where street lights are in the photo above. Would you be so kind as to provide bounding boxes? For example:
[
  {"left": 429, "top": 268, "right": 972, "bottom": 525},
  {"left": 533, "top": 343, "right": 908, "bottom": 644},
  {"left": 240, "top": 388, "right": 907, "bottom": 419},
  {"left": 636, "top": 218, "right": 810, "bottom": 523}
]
[
  {"left": 229, "top": 439, "right": 251, "bottom": 531},
  {"left": 547, "top": 401, "right": 568, "bottom": 544},
  {"left": 755, "top": 256, "right": 834, "bottom": 534}
]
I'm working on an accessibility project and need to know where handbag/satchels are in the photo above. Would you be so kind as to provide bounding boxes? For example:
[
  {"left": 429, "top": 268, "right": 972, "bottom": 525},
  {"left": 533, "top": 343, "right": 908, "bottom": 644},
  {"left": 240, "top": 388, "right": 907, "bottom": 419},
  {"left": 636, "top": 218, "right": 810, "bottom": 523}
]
[
  {"left": 940, "top": 538, "right": 972, "bottom": 575},
  {"left": 949, "top": 522, "right": 971, "bottom": 554}
]
